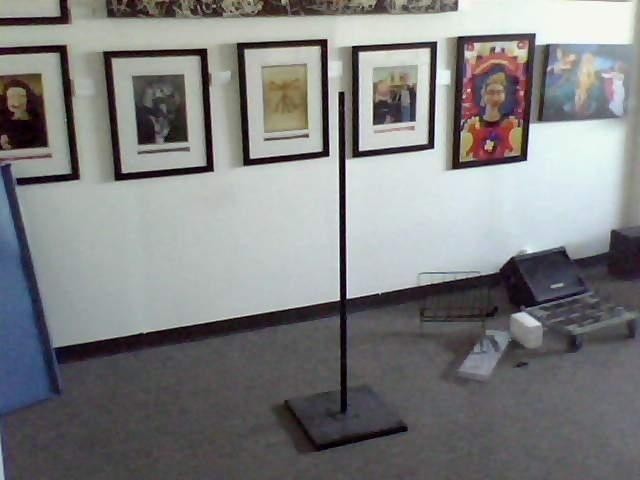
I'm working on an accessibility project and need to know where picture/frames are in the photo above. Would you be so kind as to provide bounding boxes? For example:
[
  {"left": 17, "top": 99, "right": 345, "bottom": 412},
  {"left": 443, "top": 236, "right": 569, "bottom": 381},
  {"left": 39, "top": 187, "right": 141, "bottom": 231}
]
[
  {"left": 352, "top": 41, "right": 437, "bottom": 158},
  {"left": 0, "top": 0, "right": 69, "bottom": 26},
  {"left": 236, "top": 39, "right": 329, "bottom": 165},
  {"left": 452, "top": 33, "right": 536, "bottom": 168},
  {"left": 0, "top": 45, "right": 81, "bottom": 185},
  {"left": 102, "top": 48, "right": 214, "bottom": 181}
]
